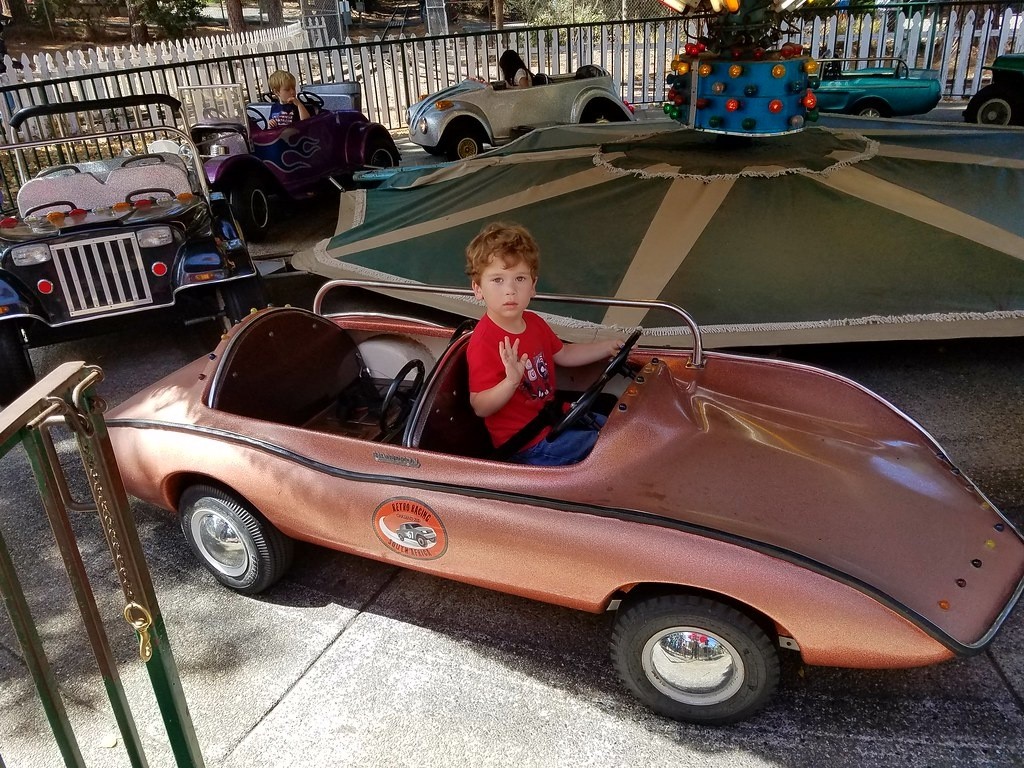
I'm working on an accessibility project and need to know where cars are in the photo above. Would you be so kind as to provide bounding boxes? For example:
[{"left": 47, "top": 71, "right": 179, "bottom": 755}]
[
  {"left": 404, "top": 61, "right": 637, "bottom": 165},
  {"left": 183, "top": 88, "right": 401, "bottom": 242},
  {"left": 98, "top": 277, "right": 1023, "bottom": 726},
  {"left": 0, "top": 90, "right": 257, "bottom": 399},
  {"left": 805, "top": 51, "right": 943, "bottom": 121},
  {"left": 962, "top": 56, "right": 1023, "bottom": 125}
]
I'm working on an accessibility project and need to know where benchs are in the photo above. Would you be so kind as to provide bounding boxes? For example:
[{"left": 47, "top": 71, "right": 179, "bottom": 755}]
[{"left": 16, "top": 163, "right": 192, "bottom": 217}]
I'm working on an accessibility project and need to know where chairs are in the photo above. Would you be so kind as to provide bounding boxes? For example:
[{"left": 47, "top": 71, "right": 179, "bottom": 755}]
[
  {"left": 407, "top": 327, "right": 619, "bottom": 466},
  {"left": 202, "top": 305, "right": 427, "bottom": 445}
]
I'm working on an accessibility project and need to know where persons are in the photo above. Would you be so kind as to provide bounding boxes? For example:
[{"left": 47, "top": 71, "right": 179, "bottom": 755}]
[
  {"left": 264, "top": 69, "right": 311, "bottom": 128},
  {"left": 499, "top": 49, "right": 533, "bottom": 90},
  {"left": 465, "top": 221, "right": 637, "bottom": 472}
]
